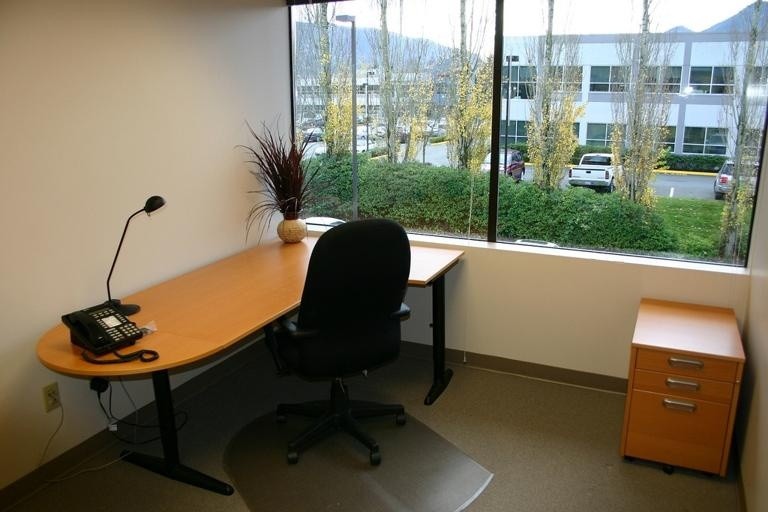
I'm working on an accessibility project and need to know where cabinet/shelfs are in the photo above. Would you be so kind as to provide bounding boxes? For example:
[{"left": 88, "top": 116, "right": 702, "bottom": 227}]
[{"left": 621, "top": 298, "right": 749, "bottom": 480}]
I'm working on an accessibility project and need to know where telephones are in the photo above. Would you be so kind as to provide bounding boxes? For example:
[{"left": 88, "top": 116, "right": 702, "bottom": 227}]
[{"left": 61, "top": 303, "right": 142, "bottom": 355}]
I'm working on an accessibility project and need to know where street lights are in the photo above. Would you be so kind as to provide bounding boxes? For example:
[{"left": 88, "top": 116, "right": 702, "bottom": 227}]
[
  {"left": 504, "top": 56, "right": 519, "bottom": 173},
  {"left": 335, "top": 15, "right": 358, "bottom": 220},
  {"left": 367, "top": 71, "right": 375, "bottom": 151}
]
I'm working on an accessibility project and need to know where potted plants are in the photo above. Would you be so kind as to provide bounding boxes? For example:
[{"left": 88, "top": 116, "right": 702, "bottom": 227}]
[{"left": 237, "top": 113, "right": 328, "bottom": 246}]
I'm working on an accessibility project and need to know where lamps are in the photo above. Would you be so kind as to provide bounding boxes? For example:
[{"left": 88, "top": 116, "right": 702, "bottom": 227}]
[{"left": 104, "top": 196, "right": 167, "bottom": 317}]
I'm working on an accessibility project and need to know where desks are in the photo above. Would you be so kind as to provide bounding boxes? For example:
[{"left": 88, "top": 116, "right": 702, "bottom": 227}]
[{"left": 35, "top": 230, "right": 467, "bottom": 494}]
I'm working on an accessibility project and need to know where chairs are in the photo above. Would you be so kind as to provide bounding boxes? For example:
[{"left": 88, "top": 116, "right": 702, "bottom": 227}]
[{"left": 257, "top": 217, "right": 414, "bottom": 466}]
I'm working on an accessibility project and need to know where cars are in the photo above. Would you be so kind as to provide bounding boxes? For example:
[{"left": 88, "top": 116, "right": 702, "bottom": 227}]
[
  {"left": 714, "top": 159, "right": 760, "bottom": 200},
  {"left": 481, "top": 148, "right": 526, "bottom": 183}
]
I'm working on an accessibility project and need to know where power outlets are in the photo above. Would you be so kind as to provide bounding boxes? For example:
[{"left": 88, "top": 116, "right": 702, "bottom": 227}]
[{"left": 39, "top": 383, "right": 62, "bottom": 414}]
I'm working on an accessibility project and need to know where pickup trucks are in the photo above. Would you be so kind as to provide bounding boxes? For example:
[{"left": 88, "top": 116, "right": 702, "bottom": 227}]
[{"left": 569, "top": 153, "right": 624, "bottom": 193}]
